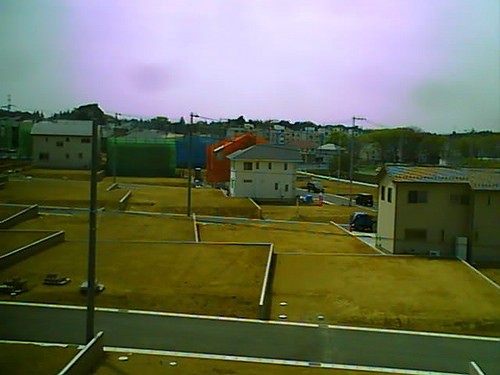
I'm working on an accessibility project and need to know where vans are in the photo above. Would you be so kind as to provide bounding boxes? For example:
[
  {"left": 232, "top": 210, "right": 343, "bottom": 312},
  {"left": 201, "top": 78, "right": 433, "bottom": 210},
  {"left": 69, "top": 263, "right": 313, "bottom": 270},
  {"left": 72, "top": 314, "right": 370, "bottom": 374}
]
[
  {"left": 354, "top": 192, "right": 374, "bottom": 207},
  {"left": 307, "top": 181, "right": 324, "bottom": 193}
]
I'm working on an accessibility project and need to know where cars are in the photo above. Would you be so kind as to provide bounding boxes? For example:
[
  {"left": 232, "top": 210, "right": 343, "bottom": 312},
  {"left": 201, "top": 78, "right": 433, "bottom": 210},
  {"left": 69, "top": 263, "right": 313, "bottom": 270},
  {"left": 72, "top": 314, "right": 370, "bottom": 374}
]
[{"left": 350, "top": 212, "right": 372, "bottom": 232}]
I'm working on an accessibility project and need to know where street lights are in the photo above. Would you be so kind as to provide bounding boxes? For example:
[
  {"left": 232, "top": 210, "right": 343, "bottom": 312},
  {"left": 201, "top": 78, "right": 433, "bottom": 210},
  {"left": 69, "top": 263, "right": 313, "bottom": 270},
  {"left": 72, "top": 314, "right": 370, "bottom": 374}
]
[
  {"left": 348, "top": 116, "right": 366, "bottom": 206},
  {"left": 113, "top": 112, "right": 122, "bottom": 184},
  {"left": 188, "top": 111, "right": 199, "bottom": 216}
]
